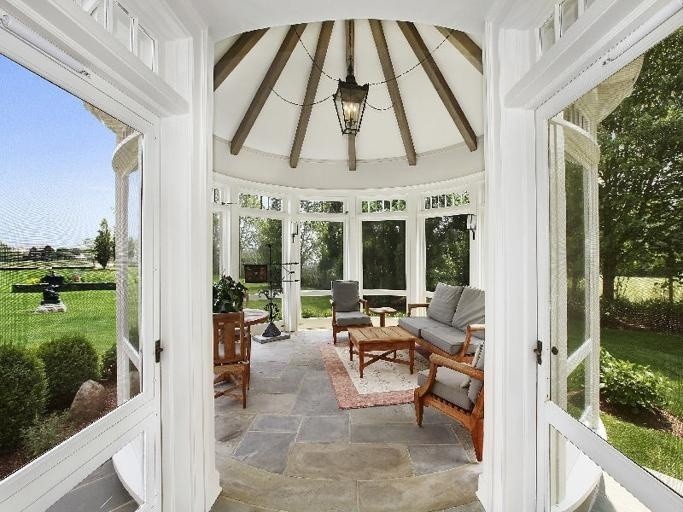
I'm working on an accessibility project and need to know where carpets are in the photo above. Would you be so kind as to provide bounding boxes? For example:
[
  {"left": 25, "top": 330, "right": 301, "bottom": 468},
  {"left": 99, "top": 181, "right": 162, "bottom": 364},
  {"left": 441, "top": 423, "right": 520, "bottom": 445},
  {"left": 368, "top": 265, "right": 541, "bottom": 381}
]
[{"left": 320, "top": 342, "right": 430, "bottom": 410}]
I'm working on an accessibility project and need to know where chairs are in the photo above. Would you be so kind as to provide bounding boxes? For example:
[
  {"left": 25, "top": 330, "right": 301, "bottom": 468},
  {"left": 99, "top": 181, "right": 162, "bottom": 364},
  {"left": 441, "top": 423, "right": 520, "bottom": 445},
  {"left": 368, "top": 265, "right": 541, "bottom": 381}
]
[
  {"left": 213, "top": 312, "right": 251, "bottom": 408},
  {"left": 414, "top": 341, "right": 485, "bottom": 461},
  {"left": 328, "top": 279, "right": 373, "bottom": 345}
]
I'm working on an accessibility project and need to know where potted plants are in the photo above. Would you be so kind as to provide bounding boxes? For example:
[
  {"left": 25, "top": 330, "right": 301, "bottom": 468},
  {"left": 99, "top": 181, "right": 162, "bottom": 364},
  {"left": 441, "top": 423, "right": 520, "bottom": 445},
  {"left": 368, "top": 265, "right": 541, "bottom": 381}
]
[{"left": 213, "top": 275, "right": 248, "bottom": 313}]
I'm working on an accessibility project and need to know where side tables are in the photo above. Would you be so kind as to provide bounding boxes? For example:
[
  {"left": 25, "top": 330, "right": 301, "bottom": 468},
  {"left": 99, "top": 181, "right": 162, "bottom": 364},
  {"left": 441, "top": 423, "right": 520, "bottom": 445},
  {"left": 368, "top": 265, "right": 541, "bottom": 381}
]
[{"left": 370, "top": 306, "right": 397, "bottom": 327}]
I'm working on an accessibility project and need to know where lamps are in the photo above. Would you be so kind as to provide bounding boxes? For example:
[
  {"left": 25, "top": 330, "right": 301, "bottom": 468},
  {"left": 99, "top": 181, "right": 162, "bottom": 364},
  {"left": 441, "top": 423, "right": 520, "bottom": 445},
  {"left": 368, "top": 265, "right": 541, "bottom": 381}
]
[
  {"left": 291, "top": 223, "right": 299, "bottom": 243},
  {"left": 467, "top": 214, "right": 477, "bottom": 240},
  {"left": 331, "top": 20, "right": 370, "bottom": 137}
]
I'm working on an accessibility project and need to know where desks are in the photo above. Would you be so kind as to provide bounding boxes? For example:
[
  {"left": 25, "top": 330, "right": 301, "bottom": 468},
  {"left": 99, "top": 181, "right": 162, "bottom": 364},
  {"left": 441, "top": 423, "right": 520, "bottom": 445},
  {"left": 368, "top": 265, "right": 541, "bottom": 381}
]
[{"left": 214, "top": 307, "right": 269, "bottom": 384}]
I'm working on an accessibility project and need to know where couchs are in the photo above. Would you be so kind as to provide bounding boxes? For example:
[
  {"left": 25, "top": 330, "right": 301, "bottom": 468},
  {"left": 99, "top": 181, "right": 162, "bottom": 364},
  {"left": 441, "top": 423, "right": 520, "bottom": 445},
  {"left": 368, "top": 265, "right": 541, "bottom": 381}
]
[{"left": 396, "top": 282, "right": 485, "bottom": 367}]
